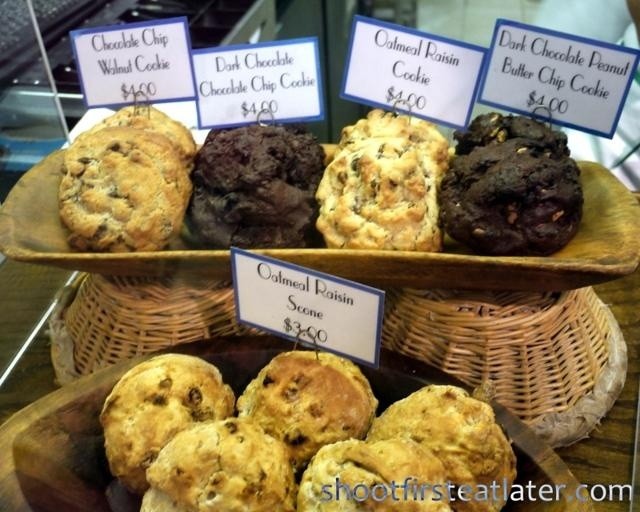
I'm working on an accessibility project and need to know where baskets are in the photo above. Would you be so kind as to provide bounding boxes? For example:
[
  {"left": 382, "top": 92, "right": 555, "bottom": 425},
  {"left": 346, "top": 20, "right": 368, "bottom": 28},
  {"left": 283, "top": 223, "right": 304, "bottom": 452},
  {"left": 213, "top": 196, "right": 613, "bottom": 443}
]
[{"left": 47, "top": 271, "right": 630, "bottom": 448}]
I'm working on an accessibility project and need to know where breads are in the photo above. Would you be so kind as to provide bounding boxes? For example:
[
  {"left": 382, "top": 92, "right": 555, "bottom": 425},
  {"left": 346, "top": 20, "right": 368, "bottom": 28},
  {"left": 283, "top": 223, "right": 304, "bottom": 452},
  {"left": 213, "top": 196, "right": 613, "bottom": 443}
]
[
  {"left": 60, "top": 102, "right": 584, "bottom": 255},
  {"left": 58, "top": 350, "right": 586, "bottom": 512}
]
